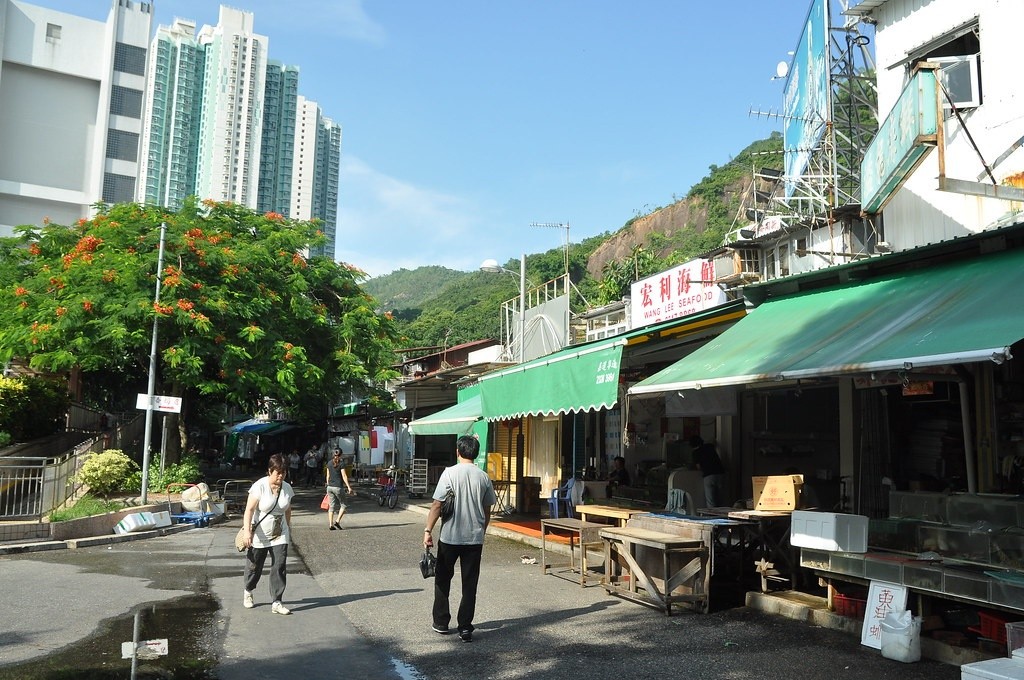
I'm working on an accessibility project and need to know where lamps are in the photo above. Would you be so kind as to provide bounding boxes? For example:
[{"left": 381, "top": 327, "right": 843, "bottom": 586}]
[
  {"left": 759, "top": 168, "right": 782, "bottom": 183},
  {"left": 746, "top": 209, "right": 762, "bottom": 222},
  {"left": 752, "top": 190, "right": 770, "bottom": 203},
  {"left": 739, "top": 230, "right": 754, "bottom": 240}
]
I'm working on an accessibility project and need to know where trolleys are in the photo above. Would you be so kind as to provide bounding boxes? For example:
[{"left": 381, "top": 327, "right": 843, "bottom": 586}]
[
  {"left": 166, "top": 483, "right": 225, "bottom": 528},
  {"left": 404, "top": 458, "right": 428, "bottom": 498},
  {"left": 215, "top": 478, "right": 253, "bottom": 515}
]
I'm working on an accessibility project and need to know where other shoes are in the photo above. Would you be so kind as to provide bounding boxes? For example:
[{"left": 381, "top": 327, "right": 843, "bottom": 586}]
[
  {"left": 334, "top": 521, "right": 342, "bottom": 530},
  {"left": 330, "top": 526, "right": 336, "bottom": 530}
]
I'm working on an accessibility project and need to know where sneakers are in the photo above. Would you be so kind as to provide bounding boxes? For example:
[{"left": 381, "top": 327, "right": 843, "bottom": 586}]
[
  {"left": 244, "top": 589, "right": 254, "bottom": 608},
  {"left": 432, "top": 623, "right": 449, "bottom": 633},
  {"left": 459, "top": 629, "right": 472, "bottom": 643},
  {"left": 272, "top": 602, "right": 290, "bottom": 614}
]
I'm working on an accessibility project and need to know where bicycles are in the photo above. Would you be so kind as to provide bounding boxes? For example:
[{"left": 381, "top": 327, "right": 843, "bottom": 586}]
[{"left": 377, "top": 468, "right": 410, "bottom": 509}]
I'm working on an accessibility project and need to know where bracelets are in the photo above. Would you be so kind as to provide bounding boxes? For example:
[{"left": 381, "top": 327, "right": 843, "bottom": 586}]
[{"left": 424, "top": 528, "right": 431, "bottom": 533}]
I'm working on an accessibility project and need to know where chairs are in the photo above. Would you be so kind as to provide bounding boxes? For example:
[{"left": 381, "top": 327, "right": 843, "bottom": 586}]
[{"left": 548, "top": 478, "right": 575, "bottom": 520}]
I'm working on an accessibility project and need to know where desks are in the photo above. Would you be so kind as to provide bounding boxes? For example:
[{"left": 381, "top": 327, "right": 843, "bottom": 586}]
[
  {"left": 541, "top": 519, "right": 613, "bottom": 589},
  {"left": 606, "top": 527, "right": 707, "bottom": 618},
  {"left": 576, "top": 504, "right": 649, "bottom": 574},
  {"left": 488, "top": 480, "right": 518, "bottom": 516},
  {"left": 569, "top": 480, "right": 617, "bottom": 507}
]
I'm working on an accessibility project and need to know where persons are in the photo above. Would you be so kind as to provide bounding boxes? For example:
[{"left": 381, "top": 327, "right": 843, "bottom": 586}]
[
  {"left": 326, "top": 447, "right": 354, "bottom": 530},
  {"left": 242, "top": 454, "right": 295, "bottom": 615},
  {"left": 688, "top": 434, "right": 737, "bottom": 509},
  {"left": 288, "top": 449, "right": 300, "bottom": 487},
  {"left": 605, "top": 456, "right": 631, "bottom": 498},
  {"left": 782, "top": 466, "right": 820, "bottom": 505},
  {"left": 305, "top": 445, "right": 322, "bottom": 489},
  {"left": 422, "top": 435, "right": 498, "bottom": 643}
]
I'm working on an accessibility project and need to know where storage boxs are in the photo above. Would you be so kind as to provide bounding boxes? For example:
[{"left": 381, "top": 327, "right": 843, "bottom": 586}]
[
  {"left": 752, "top": 465, "right": 1024, "bottom": 680},
  {"left": 114, "top": 509, "right": 172, "bottom": 536},
  {"left": 206, "top": 491, "right": 228, "bottom": 514}
]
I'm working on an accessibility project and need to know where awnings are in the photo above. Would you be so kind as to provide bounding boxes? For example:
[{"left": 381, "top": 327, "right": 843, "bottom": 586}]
[
  {"left": 626, "top": 244, "right": 1024, "bottom": 400},
  {"left": 408, "top": 395, "right": 484, "bottom": 435},
  {"left": 477, "top": 297, "right": 746, "bottom": 423}
]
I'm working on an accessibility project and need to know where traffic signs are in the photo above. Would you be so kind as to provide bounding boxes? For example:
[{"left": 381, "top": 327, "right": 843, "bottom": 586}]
[
  {"left": 120, "top": 638, "right": 169, "bottom": 660},
  {"left": 135, "top": 393, "right": 182, "bottom": 414}
]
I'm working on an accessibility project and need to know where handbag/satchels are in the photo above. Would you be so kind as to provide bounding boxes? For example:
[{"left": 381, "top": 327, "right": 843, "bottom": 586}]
[
  {"left": 321, "top": 494, "right": 329, "bottom": 511},
  {"left": 419, "top": 544, "right": 438, "bottom": 578},
  {"left": 234, "top": 523, "right": 254, "bottom": 552},
  {"left": 440, "top": 489, "right": 455, "bottom": 517}
]
